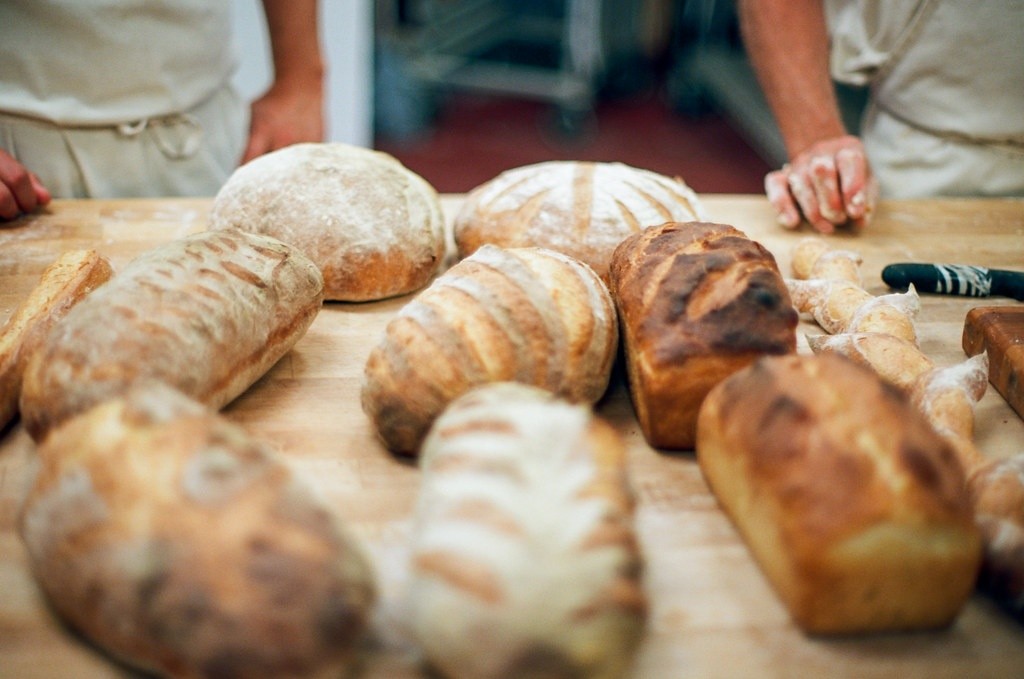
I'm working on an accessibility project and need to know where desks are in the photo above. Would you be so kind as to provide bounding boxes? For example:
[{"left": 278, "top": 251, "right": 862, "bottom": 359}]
[{"left": 0, "top": 197, "right": 1024, "bottom": 679}]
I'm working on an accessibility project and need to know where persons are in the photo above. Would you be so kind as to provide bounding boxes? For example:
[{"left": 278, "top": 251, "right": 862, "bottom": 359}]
[
  {"left": 739, "top": 0, "right": 1024, "bottom": 234},
  {"left": 0, "top": 0, "right": 328, "bottom": 223}
]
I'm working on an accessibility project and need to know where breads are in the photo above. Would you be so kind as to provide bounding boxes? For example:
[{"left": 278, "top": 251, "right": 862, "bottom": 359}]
[{"left": 0, "top": 143, "right": 1024, "bottom": 679}]
